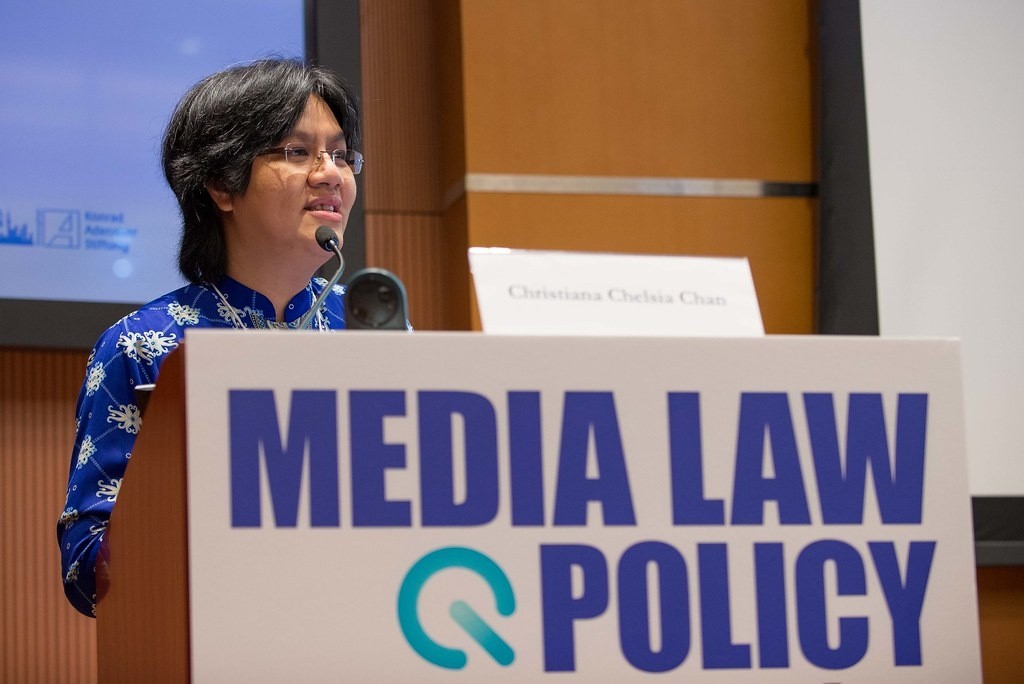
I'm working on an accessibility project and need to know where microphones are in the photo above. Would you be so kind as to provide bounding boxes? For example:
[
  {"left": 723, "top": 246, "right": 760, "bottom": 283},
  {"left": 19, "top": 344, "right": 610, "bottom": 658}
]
[{"left": 297, "top": 225, "right": 345, "bottom": 330}]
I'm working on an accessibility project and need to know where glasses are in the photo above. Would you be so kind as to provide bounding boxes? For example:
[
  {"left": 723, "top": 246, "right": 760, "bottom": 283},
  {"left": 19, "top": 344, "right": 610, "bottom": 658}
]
[{"left": 256, "top": 141, "right": 365, "bottom": 175}]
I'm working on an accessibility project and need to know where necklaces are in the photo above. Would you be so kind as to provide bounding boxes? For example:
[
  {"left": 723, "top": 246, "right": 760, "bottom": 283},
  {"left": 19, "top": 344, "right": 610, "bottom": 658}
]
[{"left": 211, "top": 284, "right": 321, "bottom": 329}]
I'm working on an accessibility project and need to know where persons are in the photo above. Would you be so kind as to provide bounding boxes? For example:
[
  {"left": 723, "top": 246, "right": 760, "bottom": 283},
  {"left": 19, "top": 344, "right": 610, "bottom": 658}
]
[{"left": 54, "top": 61, "right": 413, "bottom": 617}]
